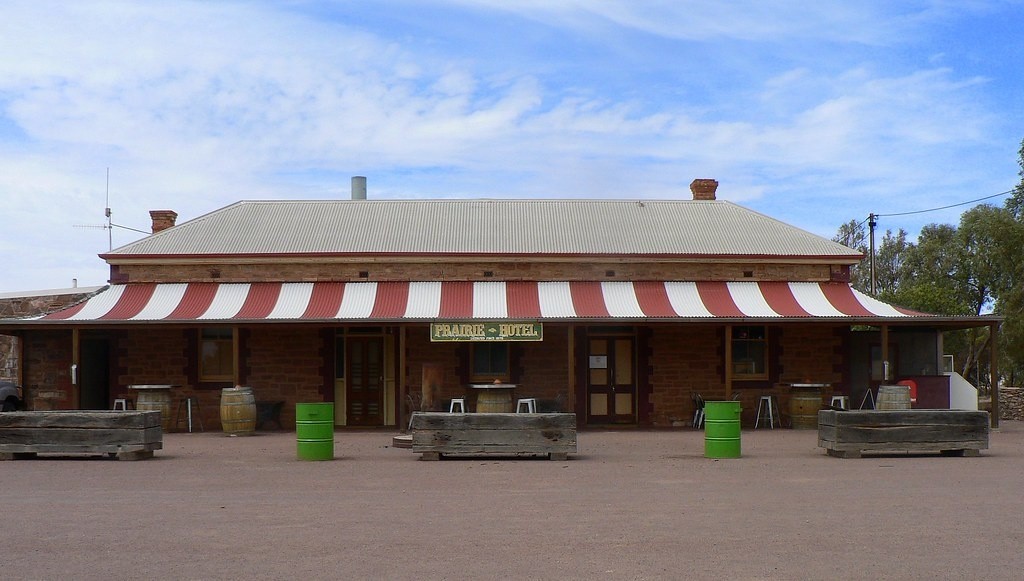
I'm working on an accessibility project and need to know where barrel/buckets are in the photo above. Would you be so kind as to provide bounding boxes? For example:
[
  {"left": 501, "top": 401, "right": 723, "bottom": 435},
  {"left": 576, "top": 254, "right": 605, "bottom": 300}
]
[
  {"left": 296, "top": 402, "right": 334, "bottom": 461},
  {"left": 220, "top": 387, "right": 256, "bottom": 436},
  {"left": 790, "top": 388, "right": 823, "bottom": 430},
  {"left": 704, "top": 401, "right": 741, "bottom": 459},
  {"left": 876, "top": 386, "right": 911, "bottom": 410},
  {"left": 476, "top": 389, "right": 512, "bottom": 413},
  {"left": 137, "top": 392, "right": 171, "bottom": 433}
]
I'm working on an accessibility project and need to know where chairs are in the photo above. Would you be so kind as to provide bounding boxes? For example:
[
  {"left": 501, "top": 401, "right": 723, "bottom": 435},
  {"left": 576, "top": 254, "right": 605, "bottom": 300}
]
[
  {"left": 689, "top": 391, "right": 744, "bottom": 429},
  {"left": 406, "top": 392, "right": 422, "bottom": 430}
]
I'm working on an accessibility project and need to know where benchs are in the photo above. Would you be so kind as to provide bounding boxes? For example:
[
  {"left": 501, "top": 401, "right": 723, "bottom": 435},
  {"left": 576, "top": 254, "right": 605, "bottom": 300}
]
[
  {"left": 412, "top": 412, "right": 577, "bottom": 461},
  {"left": 818, "top": 409, "right": 989, "bottom": 459},
  {"left": 0, "top": 408, "right": 163, "bottom": 460}
]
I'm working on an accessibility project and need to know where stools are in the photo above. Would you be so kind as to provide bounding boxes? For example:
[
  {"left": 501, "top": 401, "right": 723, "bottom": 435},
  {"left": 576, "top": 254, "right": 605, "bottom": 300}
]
[
  {"left": 177, "top": 396, "right": 204, "bottom": 433},
  {"left": 516, "top": 398, "right": 536, "bottom": 413},
  {"left": 754, "top": 395, "right": 782, "bottom": 429},
  {"left": 450, "top": 399, "right": 469, "bottom": 413},
  {"left": 112, "top": 398, "right": 134, "bottom": 410},
  {"left": 831, "top": 396, "right": 850, "bottom": 409}
]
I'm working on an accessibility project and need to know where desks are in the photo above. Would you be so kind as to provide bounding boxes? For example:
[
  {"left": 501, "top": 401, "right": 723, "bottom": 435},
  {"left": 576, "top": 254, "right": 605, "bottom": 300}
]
[
  {"left": 130, "top": 385, "right": 177, "bottom": 433},
  {"left": 784, "top": 380, "right": 832, "bottom": 429},
  {"left": 471, "top": 383, "right": 518, "bottom": 414}
]
